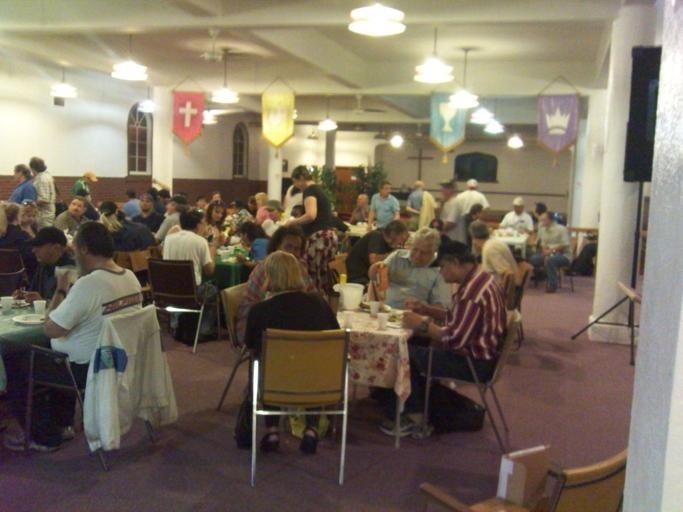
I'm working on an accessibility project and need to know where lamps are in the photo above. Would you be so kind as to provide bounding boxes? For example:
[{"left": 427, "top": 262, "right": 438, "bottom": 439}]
[
  {"left": 313, "top": 93, "right": 338, "bottom": 135},
  {"left": 108, "top": 33, "right": 148, "bottom": 83},
  {"left": 344, "top": 2, "right": 529, "bottom": 153},
  {"left": 134, "top": 88, "right": 162, "bottom": 115},
  {"left": 47, "top": 64, "right": 77, "bottom": 99},
  {"left": 207, "top": 46, "right": 239, "bottom": 105}
]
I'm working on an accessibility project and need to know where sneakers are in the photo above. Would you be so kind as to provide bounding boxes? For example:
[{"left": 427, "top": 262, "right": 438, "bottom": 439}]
[
  {"left": 4, "top": 431, "right": 60, "bottom": 452},
  {"left": 381, "top": 416, "right": 433, "bottom": 440},
  {"left": 61, "top": 427, "right": 74, "bottom": 439}
]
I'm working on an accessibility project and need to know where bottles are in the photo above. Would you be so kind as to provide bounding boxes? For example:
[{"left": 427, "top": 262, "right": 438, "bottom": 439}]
[{"left": 375, "top": 263, "right": 388, "bottom": 291}]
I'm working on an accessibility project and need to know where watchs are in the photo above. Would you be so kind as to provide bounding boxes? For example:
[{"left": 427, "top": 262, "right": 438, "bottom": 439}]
[{"left": 416, "top": 315, "right": 432, "bottom": 335}]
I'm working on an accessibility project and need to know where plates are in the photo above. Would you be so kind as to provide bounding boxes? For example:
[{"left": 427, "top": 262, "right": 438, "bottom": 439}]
[{"left": 11, "top": 313, "right": 45, "bottom": 325}]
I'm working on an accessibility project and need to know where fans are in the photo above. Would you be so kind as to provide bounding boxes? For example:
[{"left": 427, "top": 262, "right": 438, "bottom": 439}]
[{"left": 335, "top": 92, "right": 388, "bottom": 119}]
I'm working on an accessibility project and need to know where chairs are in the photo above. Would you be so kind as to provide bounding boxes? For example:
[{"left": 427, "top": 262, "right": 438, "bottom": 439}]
[
  {"left": 215, "top": 281, "right": 259, "bottom": 414},
  {"left": 20, "top": 300, "right": 168, "bottom": 473},
  {"left": 244, "top": 325, "right": 352, "bottom": 488},
  {"left": 142, "top": 256, "right": 221, "bottom": 353},
  {"left": 408, "top": 302, "right": 523, "bottom": 455},
  {"left": 413, "top": 439, "right": 630, "bottom": 510}
]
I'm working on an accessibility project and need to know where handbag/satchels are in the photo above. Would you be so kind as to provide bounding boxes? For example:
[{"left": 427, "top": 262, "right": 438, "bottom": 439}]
[{"left": 236, "top": 399, "right": 252, "bottom": 449}]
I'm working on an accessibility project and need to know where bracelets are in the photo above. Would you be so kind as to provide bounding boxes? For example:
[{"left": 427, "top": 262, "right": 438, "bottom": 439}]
[{"left": 52, "top": 287, "right": 69, "bottom": 297}]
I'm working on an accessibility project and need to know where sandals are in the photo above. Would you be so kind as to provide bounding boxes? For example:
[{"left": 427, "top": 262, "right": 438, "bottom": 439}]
[
  {"left": 299, "top": 427, "right": 318, "bottom": 453},
  {"left": 262, "top": 431, "right": 280, "bottom": 449}
]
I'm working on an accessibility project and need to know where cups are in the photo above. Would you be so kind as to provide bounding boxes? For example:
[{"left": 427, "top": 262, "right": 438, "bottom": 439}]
[
  {"left": 376, "top": 312, "right": 389, "bottom": 330},
  {"left": 327, "top": 291, "right": 338, "bottom": 317},
  {"left": 32, "top": 300, "right": 46, "bottom": 313},
  {"left": 0, "top": 297, "right": 12, "bottom": 315},
  {"left": 219, "top": 248, "right": 229, "bottom": 262},
  {"left": 368, "top": 303, "right": 379, "bottom": 317},
  {"left": 340, "top": 311, "right": 353, "bottom": 329}
]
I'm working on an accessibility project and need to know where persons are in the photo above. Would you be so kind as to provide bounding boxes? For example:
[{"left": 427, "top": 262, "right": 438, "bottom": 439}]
[
  {"left": 162, "top": 163, "right": 343, "bottom": 341},
  {"left": 374, "top": 237, "right": 507, "bottom": 440},
  {"left": 0, "top": 156, "right": 171, "bottom": 303},
  {"left": 1, "top": 221, "right": 146, "bottom": 454},
  {"left": 231, "top": 222, "right": 323, "bottom": 452},
  {"left": 240, "top": 247, "right": 343, "bottom": 456},
  {"left": 349, "top": 177, "right": 572, "bottom": 293}
]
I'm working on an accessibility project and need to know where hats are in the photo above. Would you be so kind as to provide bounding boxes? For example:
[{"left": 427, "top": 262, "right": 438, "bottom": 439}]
[
  {"left": 24, "top": 226, "right": 66, "bottom": 245},
  {"left": 84, "top": 171, "right": 96, "bottom": 182},
  {"left": 264, "top": 200, "right": 281, "bottom": 209},
  {"left": 429, "top": 240, "right": 469, "bottom": 267}
]
